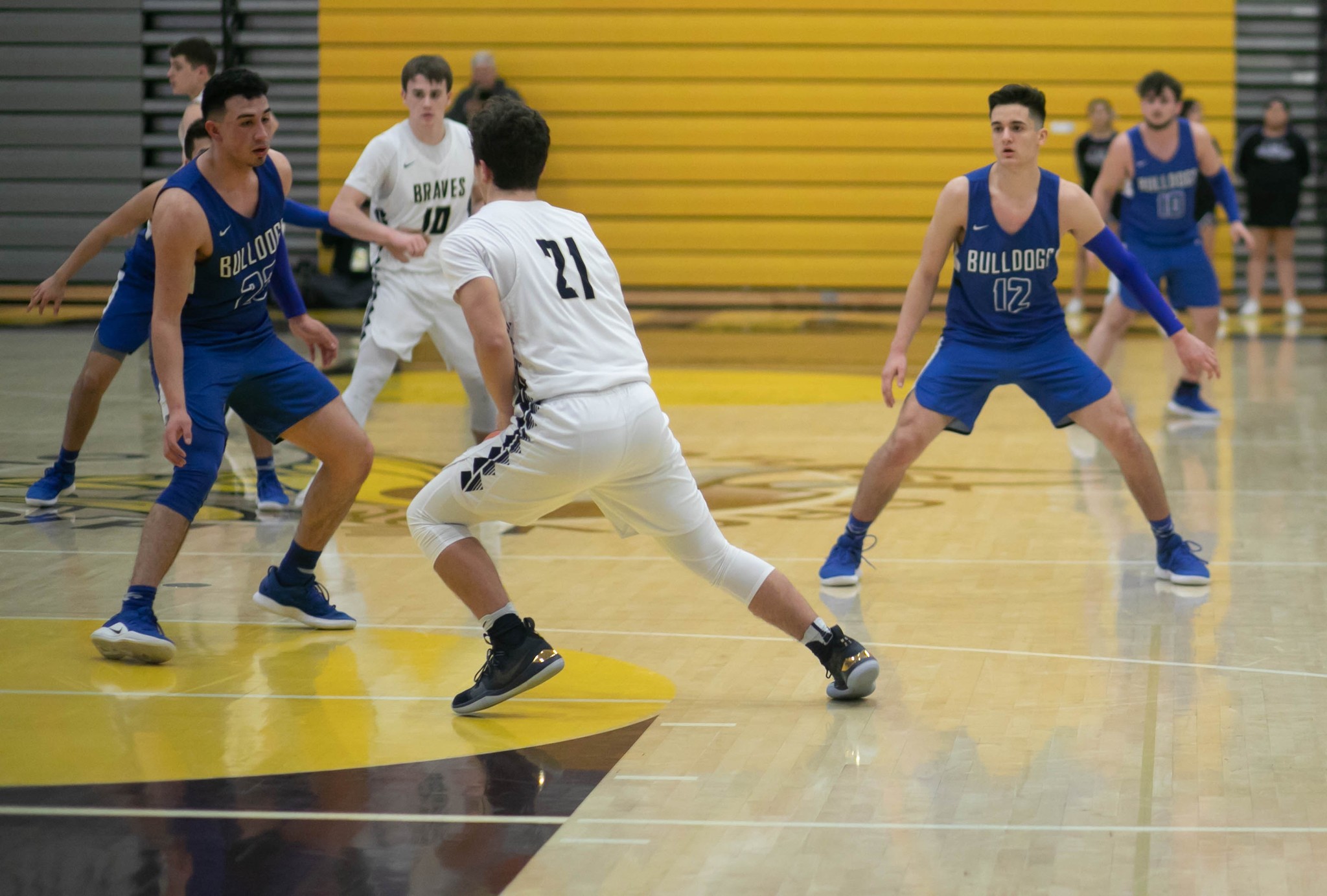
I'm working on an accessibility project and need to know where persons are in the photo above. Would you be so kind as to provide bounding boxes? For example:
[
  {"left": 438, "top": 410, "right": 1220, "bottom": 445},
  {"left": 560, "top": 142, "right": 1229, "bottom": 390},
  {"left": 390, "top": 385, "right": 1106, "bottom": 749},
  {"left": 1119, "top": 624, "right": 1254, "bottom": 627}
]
[
  {"left": 1065, "top": 71, "right": 1310, "bottom": 420},
  {"left": 404, "top": 97, "right": 878, "bottom": 716},
  {"left": 90, "top": 70, "right": 375, "bottom": 664},
  {"left": 23, "top": 36, "right": 519, "bottom": 510},
  {"left": 817, "top": 82, "right": 1222, "bottom": 586}
]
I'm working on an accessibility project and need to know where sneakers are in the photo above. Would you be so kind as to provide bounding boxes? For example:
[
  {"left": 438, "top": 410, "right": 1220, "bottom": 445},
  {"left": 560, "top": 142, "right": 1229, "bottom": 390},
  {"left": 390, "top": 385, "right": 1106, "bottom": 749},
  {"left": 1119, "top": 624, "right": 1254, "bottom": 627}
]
[
  {"left": 826, "top": 628, "right": 878, "bottom": 698},
  {"left": 818, "top": 534, "right": 862, "bottom": 586},
  {"left": 91, "top": 609, "right": 176, "bottom": 664},
  {"left": 1155, "top": 578, "right": 1209, "bottom": 613},
  {"left": 25, "top": 462, "right": 75, "bottom": 505},
  {"left": 256, "top": 477, "right": 290, "bottom": 512},
  {"left": 452, "top": 631, "right": 564, "bottom": 714},
  {"left": 251, "top": 629, "right": 354, "bottom": 686},
  {"left": 824, "top": 699, "right": 881, "bottom": 760},
  {"left": 1154, "top": 534, "right": 1210, "bottom": 586},
  {"left": 253, "top": 568, "right": 357, "bottom": 629},
  {"left": 819, "top": 586, "right": 862, "bottom": 625},
  {"left": 90, "top": 661, "right": 177, "bottom": 702},
  {"left": 1167, "top": 392, "right": 1219, "bottom": 418},
  {"left": 255, "top": 509, "right": 288, "bottom": 541},
  {"left": 1166, "top": 419, "right": 1221, "bottom": 450},
  {"left": 23, "top": 504, "right": 76, "bottom": 536},
  {"left": 454, "top": 715, "right": 561, "bottom": 796}
]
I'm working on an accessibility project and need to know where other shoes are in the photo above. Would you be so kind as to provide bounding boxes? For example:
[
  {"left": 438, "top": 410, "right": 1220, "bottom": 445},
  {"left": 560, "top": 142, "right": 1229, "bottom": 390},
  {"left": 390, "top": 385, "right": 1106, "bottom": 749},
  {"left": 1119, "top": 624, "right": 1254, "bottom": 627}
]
[
  {"left": 1237, "top": 297, "right": 1262, "bottom": 317},
  {"left": 1062, "top": 296, "right": 1085, "bottom": 314},
  {"left": 1283, "top": 300, "right": 1304, "bottom": 316}
]
